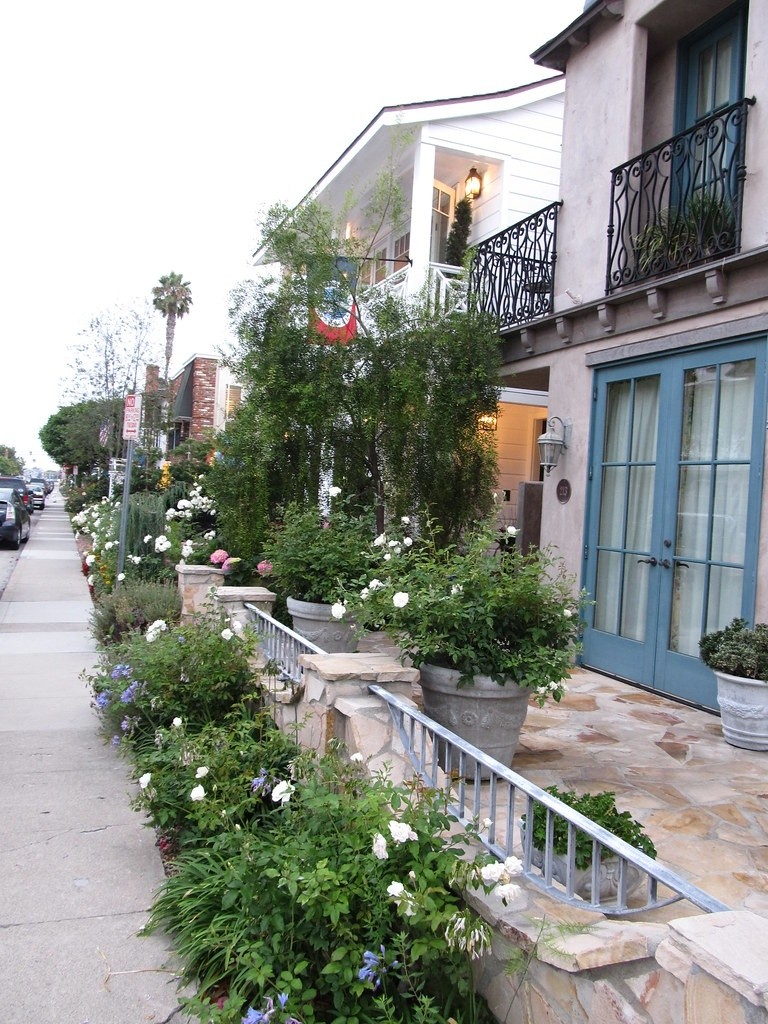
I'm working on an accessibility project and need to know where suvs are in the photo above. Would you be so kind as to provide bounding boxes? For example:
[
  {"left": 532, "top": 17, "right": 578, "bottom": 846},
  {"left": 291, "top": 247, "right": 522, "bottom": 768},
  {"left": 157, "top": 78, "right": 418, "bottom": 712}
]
[
  {"left": 0, "top": 475, "right": 34, "bottom": 513},
  {"left": 30, "top": 478, "right": 49, "bottom": 493}
]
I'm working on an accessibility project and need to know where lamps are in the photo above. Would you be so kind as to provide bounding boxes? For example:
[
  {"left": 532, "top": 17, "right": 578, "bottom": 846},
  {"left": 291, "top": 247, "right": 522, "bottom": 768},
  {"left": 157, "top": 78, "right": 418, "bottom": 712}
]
[
  {"left": 465, "top": 167, "right": 482, "bottom": 199},
  {"left": 537, "top": 416, "right": 570, "bottom": 477}
]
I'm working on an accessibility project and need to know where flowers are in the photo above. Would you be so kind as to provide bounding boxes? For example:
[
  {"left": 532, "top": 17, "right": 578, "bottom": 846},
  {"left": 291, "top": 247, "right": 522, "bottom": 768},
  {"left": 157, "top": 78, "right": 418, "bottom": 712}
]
[{"left": 67, "top": 462, "right": 596, "bottom": 1024}]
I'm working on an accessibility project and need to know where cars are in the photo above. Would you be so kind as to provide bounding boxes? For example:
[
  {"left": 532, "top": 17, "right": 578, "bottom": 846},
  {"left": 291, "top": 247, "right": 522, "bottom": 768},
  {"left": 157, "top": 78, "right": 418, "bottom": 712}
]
[
  {"left": 0, "top": 488, "right": 31, "bottom": 548},
  {"left": 30, "top": 482, "right": 46, "bottom": 493},
  {"left": 24, "top": 483, "right": 45, "bottom": 509}
]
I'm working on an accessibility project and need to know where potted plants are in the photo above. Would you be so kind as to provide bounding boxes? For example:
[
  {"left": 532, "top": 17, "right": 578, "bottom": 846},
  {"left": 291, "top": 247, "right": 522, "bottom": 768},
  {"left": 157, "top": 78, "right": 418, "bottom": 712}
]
[
  {"left": 515, "top": 786, "right": 657, "bottom": 908},
  {"left": 697, "top": 616, "right": 768, "bottom": 752}
]
